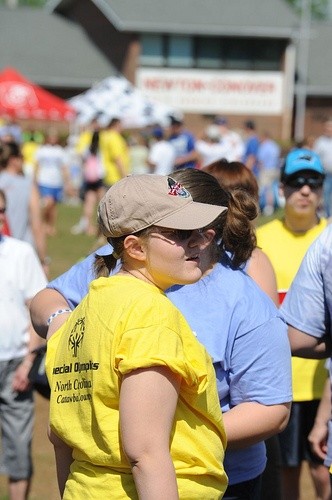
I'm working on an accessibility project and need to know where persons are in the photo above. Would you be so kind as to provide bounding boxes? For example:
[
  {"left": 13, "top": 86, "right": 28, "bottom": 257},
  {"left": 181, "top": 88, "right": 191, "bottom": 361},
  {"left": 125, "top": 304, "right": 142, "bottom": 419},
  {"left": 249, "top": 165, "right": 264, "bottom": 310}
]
[
  {"left": 307, "top": 356, "right": 331, "bottom": 467},
  {"left": 44, "top": 172, "right": 231, "bottom": 500},
  {"left": 28, "top": 166, "right": 294, "bottom": 500},
  {"left": 2, "top": 120, "right": 75, "bottom": 274},
  {"left": 254, "top": 149, "right": 331, "bottom": 500},
  {"left": 201, "top": 159, "right": 280, "bottom": 308},
  {"left": 0, "top": 189, "right": 50, "bottom": 499},
  {"left": 275, "top": 221, "right": 332, "bottom": 357},
  {"left": 73, "top": 110, "right": 331, "bottom": 229}
]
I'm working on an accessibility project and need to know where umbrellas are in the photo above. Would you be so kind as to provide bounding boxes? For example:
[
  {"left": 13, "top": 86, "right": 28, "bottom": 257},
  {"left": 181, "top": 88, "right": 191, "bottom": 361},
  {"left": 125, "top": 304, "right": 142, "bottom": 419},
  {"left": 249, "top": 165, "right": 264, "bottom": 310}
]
[
  {"left": 1, "top": 68, "right": 75, "bottom": 121},
  {"left": 66, "top": 73, "right": 182, "bottom": 128}
]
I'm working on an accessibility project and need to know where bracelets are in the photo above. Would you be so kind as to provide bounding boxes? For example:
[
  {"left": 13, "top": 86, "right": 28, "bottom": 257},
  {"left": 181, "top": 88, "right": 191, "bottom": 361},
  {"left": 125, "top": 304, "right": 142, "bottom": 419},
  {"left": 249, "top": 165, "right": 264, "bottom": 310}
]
[{"left": 45, "top": 308, "right": 74, "bottom": 336}]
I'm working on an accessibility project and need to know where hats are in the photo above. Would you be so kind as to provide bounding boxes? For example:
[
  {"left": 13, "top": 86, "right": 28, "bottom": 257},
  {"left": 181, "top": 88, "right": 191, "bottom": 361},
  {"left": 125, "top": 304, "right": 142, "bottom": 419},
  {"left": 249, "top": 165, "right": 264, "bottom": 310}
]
[
  {"left": 280, "top": 148, "right": 323, "bottom": 179},
  {"left": 98, "top": 173, "right": 229, "bottom": 237}
]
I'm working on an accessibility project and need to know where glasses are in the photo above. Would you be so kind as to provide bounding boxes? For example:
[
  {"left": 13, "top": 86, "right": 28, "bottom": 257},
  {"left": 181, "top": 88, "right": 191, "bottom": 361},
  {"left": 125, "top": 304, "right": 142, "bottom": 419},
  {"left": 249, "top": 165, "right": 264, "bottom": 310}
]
[
  {"left": 139, "top": 228, "right": 196, "bottom": 241},
  {"left": 285, "top": 176, "right": 325, "bottom": 189}
]
